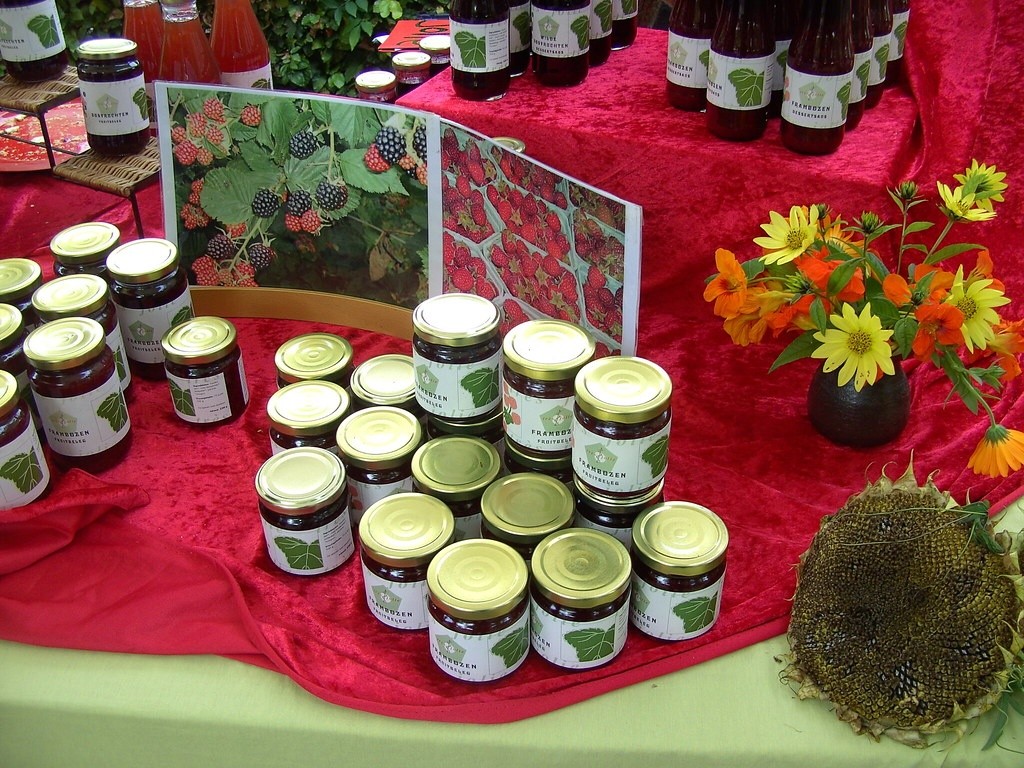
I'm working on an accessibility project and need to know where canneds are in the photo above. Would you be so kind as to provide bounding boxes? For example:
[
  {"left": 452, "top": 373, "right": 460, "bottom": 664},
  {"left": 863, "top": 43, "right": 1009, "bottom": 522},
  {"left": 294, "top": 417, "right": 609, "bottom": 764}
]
[
  {"left": 256, "top": 293, "right": 729, "bottom": 682},
  {"left": 0, "top": 0, "right": 68, "bottom": 84},
  {"left": 76, "top": 38, "right": 151, "bottom": 154},
  {"left": 0, "top": 221, "right": 248, "bottom": 510}
]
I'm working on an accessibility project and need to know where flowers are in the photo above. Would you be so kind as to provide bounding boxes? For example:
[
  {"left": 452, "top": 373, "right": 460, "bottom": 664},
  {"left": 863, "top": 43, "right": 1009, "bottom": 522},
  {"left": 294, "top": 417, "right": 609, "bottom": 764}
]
[{"left": 702, "top": 158, "right": 1024, "bottom": 479}]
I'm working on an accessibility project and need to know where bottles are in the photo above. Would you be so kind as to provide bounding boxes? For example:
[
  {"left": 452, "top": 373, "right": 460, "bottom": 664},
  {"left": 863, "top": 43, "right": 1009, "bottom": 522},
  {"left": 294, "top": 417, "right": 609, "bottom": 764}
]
[
  {"left": 356, "top": 0, "right": 638, "bottom": 103},
  {"left": 667, "top": 0, "right": 910, "bottom": 156},
  {"left": 121, "top": 1, "right": 272, "bottom": 138}
]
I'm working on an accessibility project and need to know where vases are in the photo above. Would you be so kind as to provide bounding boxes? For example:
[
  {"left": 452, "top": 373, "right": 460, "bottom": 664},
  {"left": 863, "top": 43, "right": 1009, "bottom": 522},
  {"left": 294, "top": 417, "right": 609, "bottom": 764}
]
[{"left": 806, "top": 348, "right": 915, "bottom": 450}]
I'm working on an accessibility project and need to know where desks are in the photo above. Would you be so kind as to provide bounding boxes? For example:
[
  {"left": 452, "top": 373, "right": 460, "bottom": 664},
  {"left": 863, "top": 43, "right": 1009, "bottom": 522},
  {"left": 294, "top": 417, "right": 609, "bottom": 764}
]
[{"left": 0, "top": 0, "right": 1024, "bottom": 768}]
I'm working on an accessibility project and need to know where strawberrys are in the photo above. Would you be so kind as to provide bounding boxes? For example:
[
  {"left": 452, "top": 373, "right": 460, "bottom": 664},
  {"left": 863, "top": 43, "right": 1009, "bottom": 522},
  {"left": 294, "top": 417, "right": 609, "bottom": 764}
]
[{"left": 441, "top": 128, "right": 625, "bottom": 358}]
